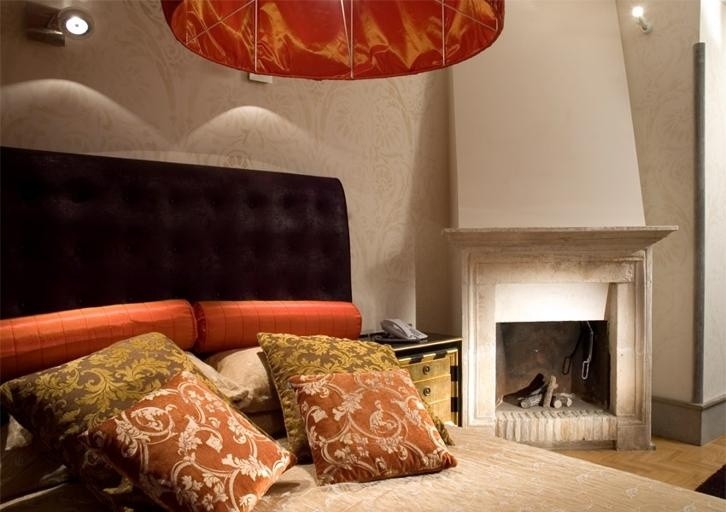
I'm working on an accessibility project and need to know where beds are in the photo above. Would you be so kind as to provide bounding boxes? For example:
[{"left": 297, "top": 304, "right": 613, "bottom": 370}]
[{"left": 0, "top": 143, "right": 726, "bottom": 512}]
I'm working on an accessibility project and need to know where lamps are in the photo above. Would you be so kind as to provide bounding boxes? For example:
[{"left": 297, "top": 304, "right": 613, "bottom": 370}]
[
  {"left": 162, "top": 0, "right": 506, "bottom": 79},
  {"left": 632, "top": 6, "right": 651, "bottom": 33},
  {"left": 25, "top": 0, "right": 95, "bottom": 46}
]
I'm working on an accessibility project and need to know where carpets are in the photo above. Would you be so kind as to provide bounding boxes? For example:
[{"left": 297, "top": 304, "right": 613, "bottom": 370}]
[{"left": 695, "top": 464, "right": 726, "bottom": 499}]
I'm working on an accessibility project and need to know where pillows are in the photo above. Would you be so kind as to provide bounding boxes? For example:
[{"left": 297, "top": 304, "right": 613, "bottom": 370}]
[
  {"left": 3, "top": 301, "right": 198, "bottom": 379},
  {"left": 191, "top": 301, "right": 362, "bottom": 354},
  {"left": 288, "top": 372, "right": 454, "bottom": 485},
  {"left": 3, "top": 333, "right": 232, "bottom": 487},
  {"left": 257, "top": 329, "right": 457, "bottom": 464},
  {"left": 209, "top": 343, "right": 279, "bottom": 409},
  {"left": 91, "top": 371, "right": 297, "bottom": 512}
]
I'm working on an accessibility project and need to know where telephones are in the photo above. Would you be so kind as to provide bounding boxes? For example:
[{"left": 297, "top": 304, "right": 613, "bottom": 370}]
[{"left": 380, "top": 318, "right": 429, "bottom": 340}]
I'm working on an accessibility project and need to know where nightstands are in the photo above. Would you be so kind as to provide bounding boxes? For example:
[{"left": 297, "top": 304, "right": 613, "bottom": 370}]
[{"left": 362, "top": 332, "right": 462, "bottom": 424}]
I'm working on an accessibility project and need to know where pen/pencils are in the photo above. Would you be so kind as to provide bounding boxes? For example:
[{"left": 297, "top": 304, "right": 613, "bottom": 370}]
[{"left": 376, "top": 338, "right": 420, "bottom": 343}]
